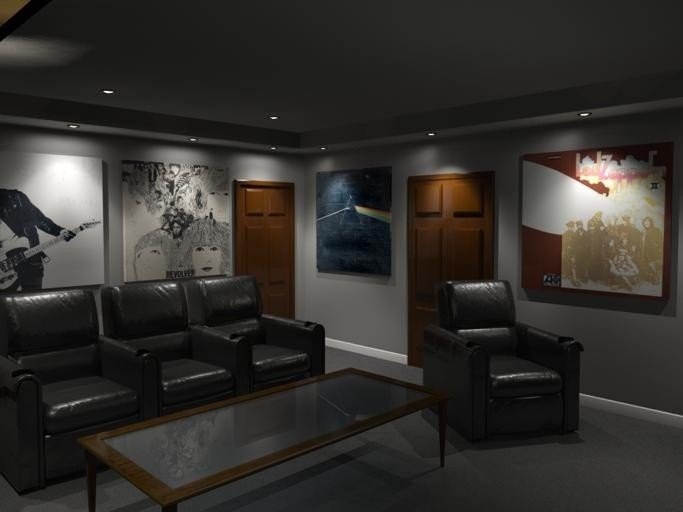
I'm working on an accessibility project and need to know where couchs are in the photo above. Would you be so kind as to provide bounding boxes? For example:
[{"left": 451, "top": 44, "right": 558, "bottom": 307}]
[{"left": 0, "top": 276, "right": 325, "bottom": 493}]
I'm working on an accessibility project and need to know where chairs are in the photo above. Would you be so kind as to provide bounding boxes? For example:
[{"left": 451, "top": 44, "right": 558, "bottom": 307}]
[{"left": 424, "top": 281, "right": 584, "bottom": 444}]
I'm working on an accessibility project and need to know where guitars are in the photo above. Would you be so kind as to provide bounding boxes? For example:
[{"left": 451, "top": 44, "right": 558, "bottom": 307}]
[{"left": 0, "top": 219, "right": 101, "bottom": 293}]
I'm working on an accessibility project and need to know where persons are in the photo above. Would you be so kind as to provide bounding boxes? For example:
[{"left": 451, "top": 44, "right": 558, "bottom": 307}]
[
  {"left": 562, "top": 211, "right": 662, "bottom": 290},
  {"left": 0, "top": 188, "right": 76, "bottom": 293},
  {"left": 123, "top": 162, "right": 230, "bottom": 282}
]
